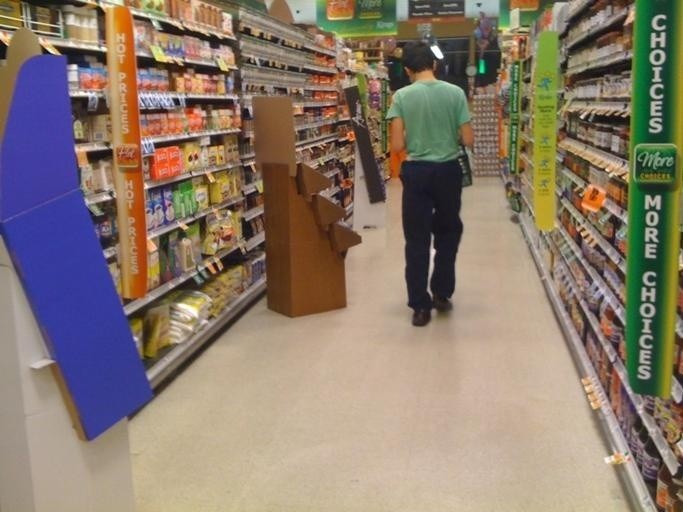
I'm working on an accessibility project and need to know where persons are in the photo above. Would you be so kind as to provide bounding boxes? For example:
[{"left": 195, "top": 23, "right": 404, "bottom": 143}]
[{"left": 381, "top": 39, "right": 479, "bottom": 328}]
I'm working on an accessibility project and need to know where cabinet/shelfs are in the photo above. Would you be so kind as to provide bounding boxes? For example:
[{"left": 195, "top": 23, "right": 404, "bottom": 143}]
[
  {"left": 500, "top": 0, "right": 683, "bottom": 512},
  {"left": 0, "top": 0, "right": 390, "bottom": 390}
]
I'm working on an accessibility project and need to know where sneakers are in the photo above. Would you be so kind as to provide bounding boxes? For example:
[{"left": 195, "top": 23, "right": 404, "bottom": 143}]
[
  {"left": 433, "top": 293, "right": 453, "bottom": 313},
  {"left": 413, "top": 307, "right": 431, "bottom": 327}
]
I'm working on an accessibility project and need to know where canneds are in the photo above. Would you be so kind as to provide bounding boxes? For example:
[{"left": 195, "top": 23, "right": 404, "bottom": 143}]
[
  {"left": 573, "top": 70, "right": 631, "bottom": 99},
  {"left": 566, "top": 118, "right": 630, "bottom": 160},
  {"left": 596, "top": 32, "right": 619, "bottom": 57}
]
